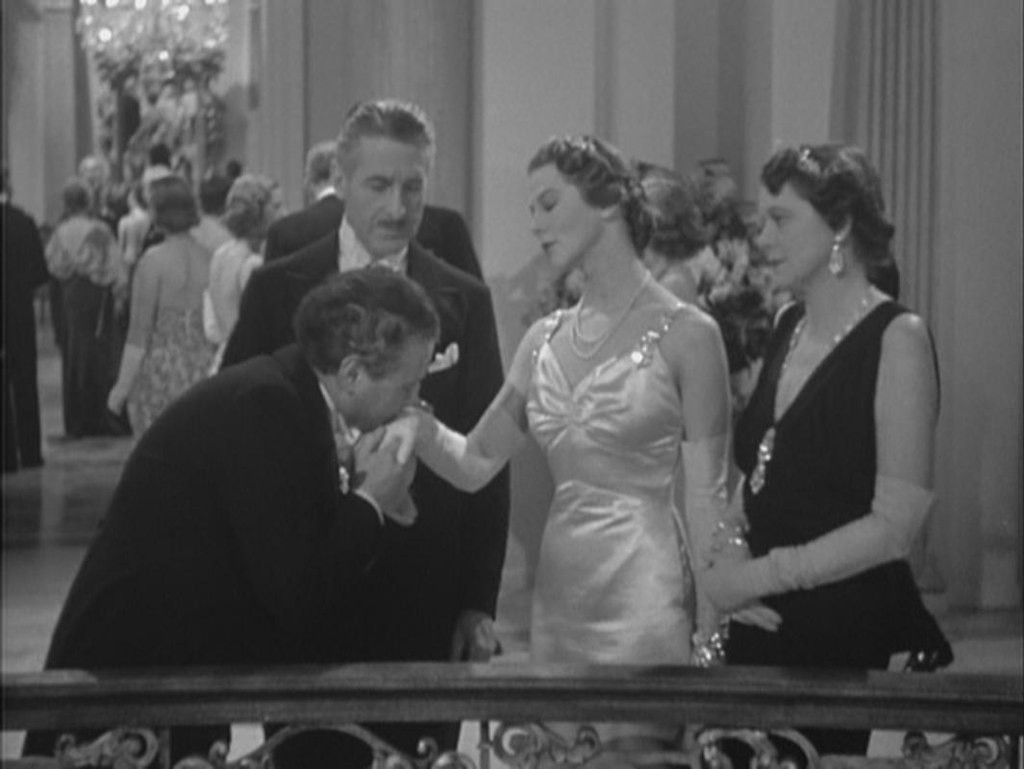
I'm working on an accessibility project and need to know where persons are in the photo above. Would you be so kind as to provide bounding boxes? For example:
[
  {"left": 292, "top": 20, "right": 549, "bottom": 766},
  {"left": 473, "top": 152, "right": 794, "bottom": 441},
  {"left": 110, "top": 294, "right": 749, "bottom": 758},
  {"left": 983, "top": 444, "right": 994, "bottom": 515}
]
[
  {"left": 0, "top": 99, "right": 511, "bottom": 769},
  {"left": 376, "top": 134, "right": 734, "bottom": 769},
  {"left": 633, "top": 157, "right": 955, "bottom": 769}
]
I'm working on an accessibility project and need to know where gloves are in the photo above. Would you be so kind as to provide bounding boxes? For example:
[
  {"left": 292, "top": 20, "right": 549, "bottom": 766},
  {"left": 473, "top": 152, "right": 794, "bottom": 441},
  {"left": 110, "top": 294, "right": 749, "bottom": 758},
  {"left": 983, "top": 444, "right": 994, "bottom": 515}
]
[{"left": 108, "top": 344, "right": 146, "bottom": 415}]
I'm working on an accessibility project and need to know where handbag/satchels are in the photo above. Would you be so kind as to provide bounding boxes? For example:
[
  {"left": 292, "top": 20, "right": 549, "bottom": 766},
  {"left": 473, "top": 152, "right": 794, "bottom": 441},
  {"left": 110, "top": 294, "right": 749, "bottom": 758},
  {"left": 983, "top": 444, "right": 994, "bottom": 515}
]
[{"left": 203, "top": 290, "right": 221, "bottom": 343}]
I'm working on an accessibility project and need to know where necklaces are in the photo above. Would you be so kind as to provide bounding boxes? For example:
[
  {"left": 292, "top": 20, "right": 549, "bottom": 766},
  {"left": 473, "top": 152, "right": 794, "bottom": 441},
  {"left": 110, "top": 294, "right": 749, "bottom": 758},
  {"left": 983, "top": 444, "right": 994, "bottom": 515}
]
[{"left": 572, "top": 272, "right": 653, "bottom": 360}]
